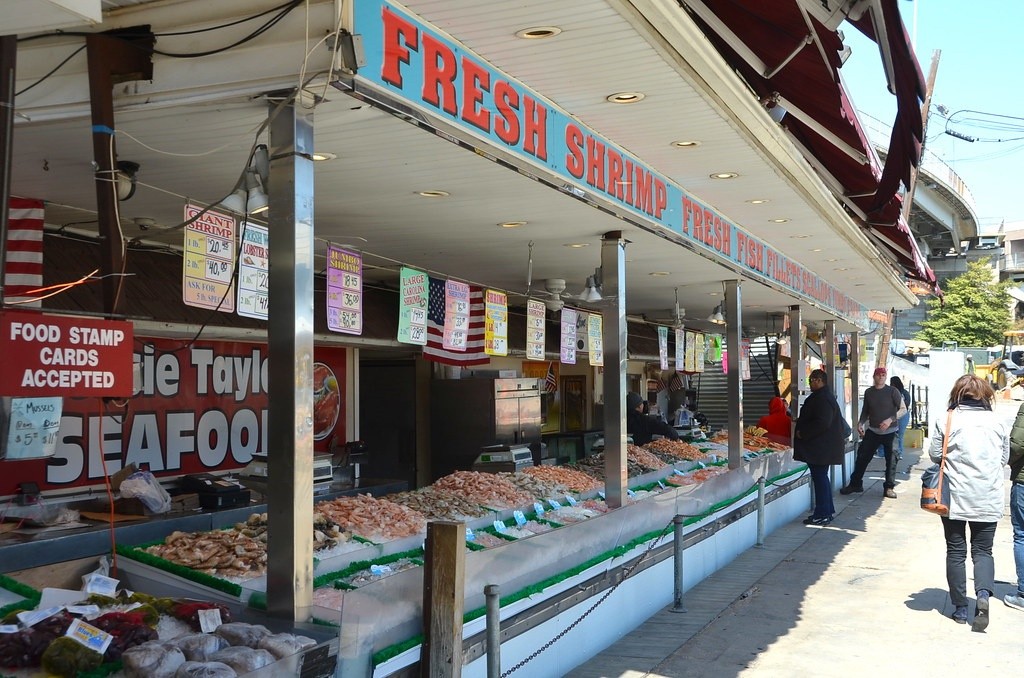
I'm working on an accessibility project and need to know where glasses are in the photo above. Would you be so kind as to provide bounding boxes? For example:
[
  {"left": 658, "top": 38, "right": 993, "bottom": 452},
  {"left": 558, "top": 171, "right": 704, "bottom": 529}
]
[{"left": 809, "top": 378, "right": 819, "bottom": 383}]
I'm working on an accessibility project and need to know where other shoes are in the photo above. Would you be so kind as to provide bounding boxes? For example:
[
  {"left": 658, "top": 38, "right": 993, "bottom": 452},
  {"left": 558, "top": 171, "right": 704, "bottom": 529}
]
[
  {"left": 883, "top": 487, "right": 897, "bottom": 498},
  {"left": 898, "top": 454, "right": 902, "bottom": 460},
  {"left": 840, "top": 484, "right": 864, "bottom": 495}
]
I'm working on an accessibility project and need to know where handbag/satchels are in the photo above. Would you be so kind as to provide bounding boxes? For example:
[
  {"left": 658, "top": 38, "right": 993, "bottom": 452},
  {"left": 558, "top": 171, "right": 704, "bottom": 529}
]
[
  {"left": 843, "top": 418, "right": 851, "bottom": 439},
  {"left": 920, "top": 464, "right": 950, "bottom": 515}
]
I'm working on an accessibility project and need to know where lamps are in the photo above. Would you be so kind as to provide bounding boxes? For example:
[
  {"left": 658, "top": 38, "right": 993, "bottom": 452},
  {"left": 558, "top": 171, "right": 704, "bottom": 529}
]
[
  {"left": 776, "top": 327, "right": 791, "bottom": 345},
  {"left": 815, "top": 329, "right": 826, "bottom": 344},
  {"left": 707, "top": 299, "right": 726, "bottom": 325},
  {"left": 837, "top": 30, "right": 851, "bottom": 65},
  {"left": 578, "top": 269, "right": 601, "bottom": 303},
  {"left": 219, "top": 147, "right": 270, "bottom": 216}
]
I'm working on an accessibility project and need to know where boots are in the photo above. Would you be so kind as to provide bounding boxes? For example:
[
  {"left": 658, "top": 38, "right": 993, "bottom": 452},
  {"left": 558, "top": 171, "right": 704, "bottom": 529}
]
[
  {"left": 952, "top": 605, "right": 968, "bottom": 624},
  {"left": 972, "top": 589, "right": 989, "bottom": 631}
]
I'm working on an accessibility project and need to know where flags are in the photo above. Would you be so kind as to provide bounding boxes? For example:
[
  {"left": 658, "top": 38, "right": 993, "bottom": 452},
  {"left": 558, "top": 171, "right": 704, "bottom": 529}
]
[
  {"left": 656, "top": 377, "right": 666, "bottom": 393},
  {"left": 670, "top": 372, "right": 682, "bottom": 392},
  {"left": 422, "top": 279, "right": 491, "bottom": 366},
  {"left": 546, "top": 363, "right": 556, "bottom": 393}
]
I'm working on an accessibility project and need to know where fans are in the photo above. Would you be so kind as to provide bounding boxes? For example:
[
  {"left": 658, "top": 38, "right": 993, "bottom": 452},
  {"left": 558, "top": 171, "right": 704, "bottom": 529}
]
[{"left": 508, "top": 278, "right": 581, "bottom": 313}]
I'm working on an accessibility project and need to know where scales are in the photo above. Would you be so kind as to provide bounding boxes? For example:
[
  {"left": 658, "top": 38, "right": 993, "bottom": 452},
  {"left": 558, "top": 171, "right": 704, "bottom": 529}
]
[
  {"left": 592, "top": 433, "right": 633, "bottom": 448},
  {"left": 239, "top": 453, "right": 334, "bottom": 485},
  {"left": 673, "top": 425, "right": 702, "bottom": 438},
  {"left": 473, "top": 443, "right": 533, "bottom": 464}
]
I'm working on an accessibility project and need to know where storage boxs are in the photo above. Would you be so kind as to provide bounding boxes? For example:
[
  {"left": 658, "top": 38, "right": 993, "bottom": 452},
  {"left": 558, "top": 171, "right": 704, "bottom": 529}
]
[{"left": 473, "top": 370, "right": 516, "bottom": 379}]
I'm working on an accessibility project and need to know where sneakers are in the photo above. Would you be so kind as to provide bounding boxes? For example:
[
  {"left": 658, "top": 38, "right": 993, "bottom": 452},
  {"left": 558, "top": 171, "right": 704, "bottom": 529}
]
[
  {"left": 803, "top": 516, "right": 830, "bottom": 525},
  {"left": 1004, "top": 592, "right": 1024, "bottom": 611},
  {"left": 808, "top": 514, "right": 833, "bottom": 521}
]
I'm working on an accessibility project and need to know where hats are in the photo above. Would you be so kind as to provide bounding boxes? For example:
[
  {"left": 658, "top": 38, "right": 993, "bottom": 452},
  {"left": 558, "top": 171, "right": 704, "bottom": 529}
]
[
  {"left": 1012, "top": 367, "right": 1024, "bottom": 375},
  {"left": 874, "top": 368, "right": 887, "bottom": 376},
  {"left": 627, "top": 391, "right": 644, "bottom": 410}
]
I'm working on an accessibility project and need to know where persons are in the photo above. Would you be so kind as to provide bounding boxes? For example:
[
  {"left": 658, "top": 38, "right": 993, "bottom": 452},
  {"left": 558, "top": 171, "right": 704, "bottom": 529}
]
[
  {"left": 964, "top": 354, "right": 976, "bottom": 375},
  {"left": 626, "top": 391, "right": 678, "bottom": 447},
  {"left": 929, "top": 374, "right": 1010, "bottom": 630},
  {"left": 1003, "top": 403, "right": 1024, "bottom": 610},
  {"left": 839, "top": 368, "right": 907, "bottom": 498},
  {"left": 878, "top": 376, "right": 911, "bottom": 460},
  {"left": 757, "top": 397, "right": 791, "bottom": 447},
  {"left": 793, "top": 369, "right": 843, "bottom": 525}
]
